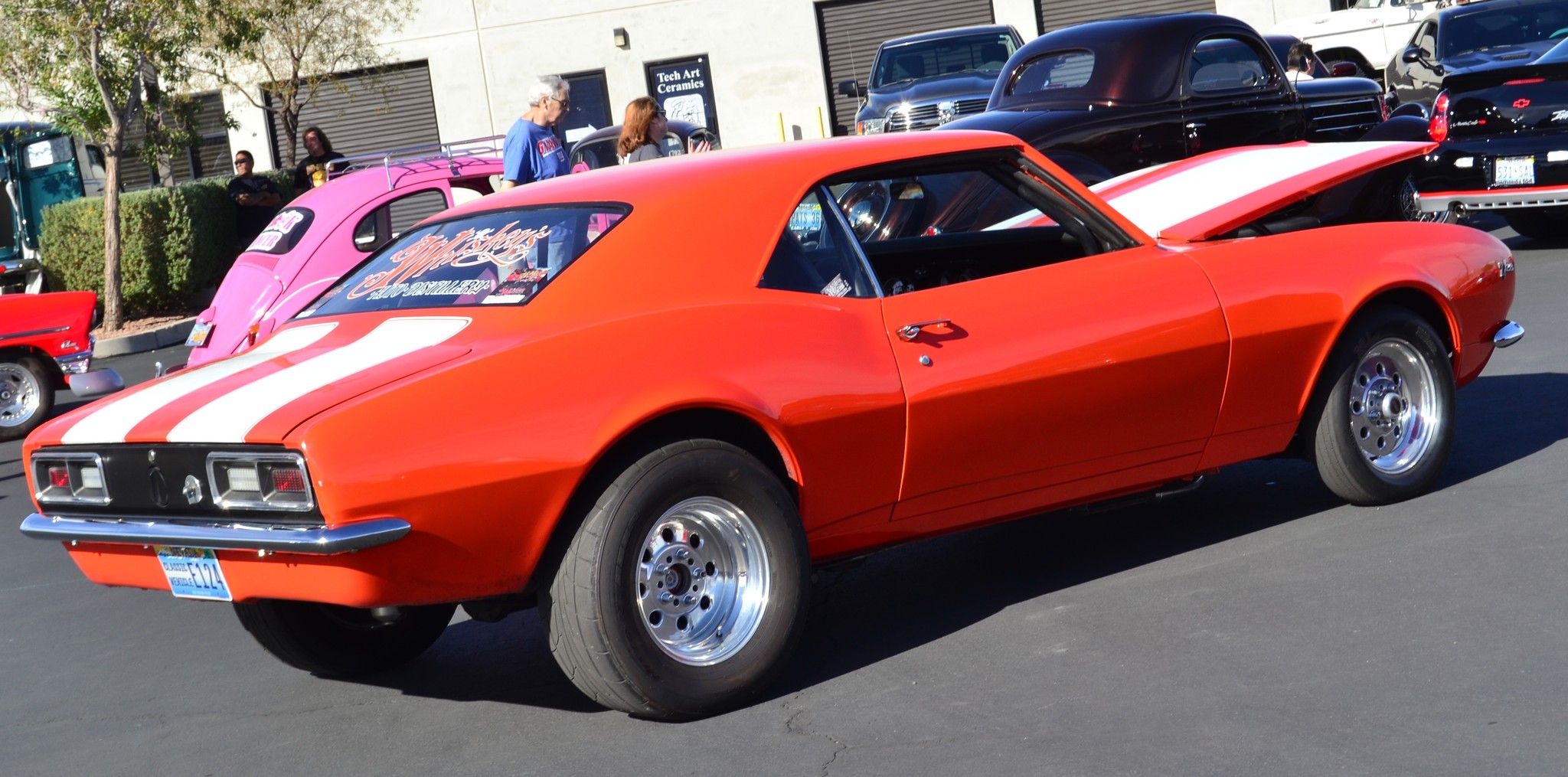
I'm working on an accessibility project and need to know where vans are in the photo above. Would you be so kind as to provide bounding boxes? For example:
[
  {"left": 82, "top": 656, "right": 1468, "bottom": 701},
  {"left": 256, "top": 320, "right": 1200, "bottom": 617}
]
[{"left": 0, "top": 119, "right": 125, "bottom": 293}]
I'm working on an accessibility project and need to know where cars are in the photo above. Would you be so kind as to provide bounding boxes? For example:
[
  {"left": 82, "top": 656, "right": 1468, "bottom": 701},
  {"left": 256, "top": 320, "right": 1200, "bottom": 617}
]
[
  {"left": 3, "top": 119, "right": 1524, "bottom": 724},
  {"left": 921, "top": 0, "right": 1568, "bottom": 244}
]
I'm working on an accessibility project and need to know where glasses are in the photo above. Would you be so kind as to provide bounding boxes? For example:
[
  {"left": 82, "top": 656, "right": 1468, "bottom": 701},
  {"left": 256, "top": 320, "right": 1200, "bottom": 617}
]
[
  {"left": 234, "top": 158, "right": 247, "bottom": 165},
  {"left": 550, "top": 97, "right": 572, "bottom": 108},
  {"left": 654, "top": 108, "right": 667, "bottom": 117}
]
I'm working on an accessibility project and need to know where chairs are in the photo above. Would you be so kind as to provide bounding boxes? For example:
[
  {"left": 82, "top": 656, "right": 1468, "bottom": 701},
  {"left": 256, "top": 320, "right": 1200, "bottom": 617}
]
[
  {"left": 980, "top": 43, "right": 1008, "bottom": 71},
  {"left": 894, "top": 54, "right": 926, "bottom": 80}
]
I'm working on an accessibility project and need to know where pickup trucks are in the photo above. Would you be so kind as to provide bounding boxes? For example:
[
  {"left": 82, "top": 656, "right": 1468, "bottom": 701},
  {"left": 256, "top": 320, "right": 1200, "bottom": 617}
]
[{"left": 841, "top": 23, "right": 1069, "bottom": 137}]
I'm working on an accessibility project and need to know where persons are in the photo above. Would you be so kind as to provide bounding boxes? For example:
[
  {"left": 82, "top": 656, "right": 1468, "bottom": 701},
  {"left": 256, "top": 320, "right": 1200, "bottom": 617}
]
[
  {"left": 500, "top": 73, "right": 590, "bottom": 291},
  {"left": 224, "top": 148, "right": 281, "bottom": 266},
  {"left": 1283, "top": 42, "right": 1316, "bottom": 84},
  {"left": 293, "top": 125, "right": 352, "bottom": 202},
  {"left": 615, "top": 95, "right": 712, "bottom": 165}
]
